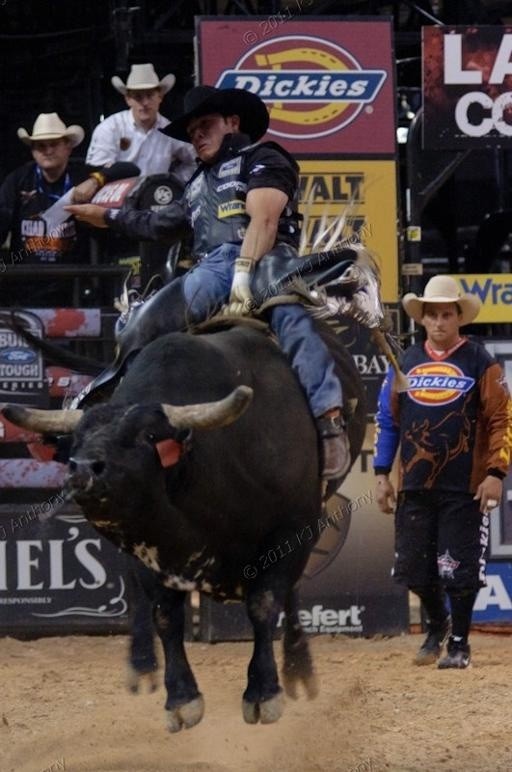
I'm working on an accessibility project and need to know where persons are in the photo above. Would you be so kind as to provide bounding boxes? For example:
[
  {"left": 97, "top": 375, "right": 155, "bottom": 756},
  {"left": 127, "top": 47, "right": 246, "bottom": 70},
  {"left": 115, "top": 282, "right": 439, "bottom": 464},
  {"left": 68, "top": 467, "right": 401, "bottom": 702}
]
[
  {"left": 367, "top": 271, "right": 512, "bottom": 674},
  {"left": 58, "top": 82, "right": 358, "bottom": 484},
  {"left": 84, "top": 61, "right": 197, "bottom": 297},
  {"left": 0, "top": 110, "right": 142, "bottom": 309}
]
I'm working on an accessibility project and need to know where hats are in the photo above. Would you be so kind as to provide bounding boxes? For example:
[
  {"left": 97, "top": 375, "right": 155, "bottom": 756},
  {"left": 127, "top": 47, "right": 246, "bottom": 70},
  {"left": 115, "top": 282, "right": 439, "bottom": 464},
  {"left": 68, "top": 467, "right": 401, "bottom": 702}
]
[
  {"left": 401, "top": 276, "right": 479, "bottom": 327},
  {"left": 16, "top": 111, "right": 85, "bottom": 148},
  {"left": 158, "top": 85, "right": 270, "bottom": 150},
  {"left": 111, "top": 63, "right": 176, "bottom": 95}
]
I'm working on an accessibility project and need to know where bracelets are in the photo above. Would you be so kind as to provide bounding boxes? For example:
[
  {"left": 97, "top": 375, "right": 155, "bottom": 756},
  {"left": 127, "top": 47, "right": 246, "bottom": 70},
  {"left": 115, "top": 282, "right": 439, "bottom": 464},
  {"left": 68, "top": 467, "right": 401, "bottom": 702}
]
[
  {"left": 375, "top": 477, "right": 390, "bottom": 486},
  {"left": 89, "top": 170, "right": 106, "bottom": 187}
]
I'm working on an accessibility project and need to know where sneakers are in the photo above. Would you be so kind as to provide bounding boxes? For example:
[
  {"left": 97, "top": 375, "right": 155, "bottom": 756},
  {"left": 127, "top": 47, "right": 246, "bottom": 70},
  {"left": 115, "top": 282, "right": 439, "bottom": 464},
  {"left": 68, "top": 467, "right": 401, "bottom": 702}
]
[
  {"left": 437, "top": 636, "right": 470, "bottom": 668},
  {"left": 416, "top": 612, "right": 452, "bottom": 661}
]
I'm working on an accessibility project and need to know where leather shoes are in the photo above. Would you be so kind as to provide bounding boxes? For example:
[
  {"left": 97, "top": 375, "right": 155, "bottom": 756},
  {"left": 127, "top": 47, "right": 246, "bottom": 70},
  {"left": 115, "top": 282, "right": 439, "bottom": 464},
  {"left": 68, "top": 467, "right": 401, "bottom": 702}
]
[{"left": 321, "top": 424, "right": 351, "bottom": 481}]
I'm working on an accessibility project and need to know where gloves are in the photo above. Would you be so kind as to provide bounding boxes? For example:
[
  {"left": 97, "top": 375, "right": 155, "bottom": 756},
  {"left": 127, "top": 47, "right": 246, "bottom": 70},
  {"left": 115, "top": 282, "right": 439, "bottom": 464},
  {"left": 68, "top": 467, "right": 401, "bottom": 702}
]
[{"left": 222, "top": 258, "right": 256, "bottom": 316}]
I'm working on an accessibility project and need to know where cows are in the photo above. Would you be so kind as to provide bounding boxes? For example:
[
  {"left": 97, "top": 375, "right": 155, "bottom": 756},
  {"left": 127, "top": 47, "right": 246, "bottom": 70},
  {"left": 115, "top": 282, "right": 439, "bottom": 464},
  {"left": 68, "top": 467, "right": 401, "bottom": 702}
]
[{"left": 1, "top": 304, "right": 370, "bottom": 734}]
